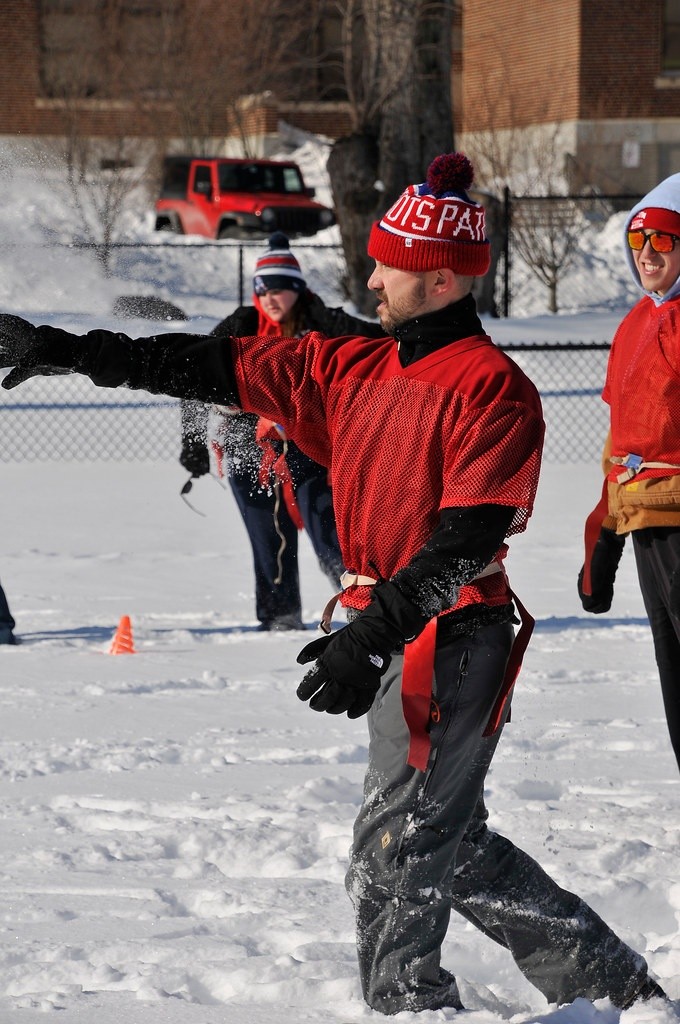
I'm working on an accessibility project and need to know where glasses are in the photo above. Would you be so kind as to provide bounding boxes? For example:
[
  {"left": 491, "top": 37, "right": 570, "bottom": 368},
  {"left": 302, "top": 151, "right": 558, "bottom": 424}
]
[
  {"left": 180, "top": 467, "right": 227, "bottom": 517},
  {"left": 626, "top": 231, "right": 680, "bottom": 254}
]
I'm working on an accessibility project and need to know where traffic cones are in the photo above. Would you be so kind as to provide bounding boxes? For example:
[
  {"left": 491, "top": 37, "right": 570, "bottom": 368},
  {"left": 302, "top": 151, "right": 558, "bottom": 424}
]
[{"left": 109, "top": 615, "right": 135, "bottom": 655}]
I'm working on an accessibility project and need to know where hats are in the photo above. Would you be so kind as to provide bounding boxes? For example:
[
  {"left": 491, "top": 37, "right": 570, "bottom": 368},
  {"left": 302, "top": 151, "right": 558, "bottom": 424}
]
[
  {"left": 367, "top": 154, "right": 491, "bottom": 277},
  {"left": 254, "top": 232, "right": 306, "bottom": 297},
  {"left": 628, "top": 208, "right": 680, "bottom": 238}
]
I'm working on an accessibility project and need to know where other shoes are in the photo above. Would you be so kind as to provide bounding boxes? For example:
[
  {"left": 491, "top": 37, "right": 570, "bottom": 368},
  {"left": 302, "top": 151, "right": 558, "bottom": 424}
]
[
  {"left": 262, "top": 613, "right": 305, "bottom": 631},
  {"left": 621, "top": 973, "right": 666, "bottom": 1011},
  {"left": 0, "top": 626, "right": 15, "bottom": 644}
]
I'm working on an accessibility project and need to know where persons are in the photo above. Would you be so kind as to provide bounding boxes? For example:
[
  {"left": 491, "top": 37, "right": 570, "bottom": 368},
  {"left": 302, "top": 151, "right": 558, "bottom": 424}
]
[
  {"left": 181, "top": 232, "right": 385, "bottom": 632},
  {"left": 576, "top": 170, "right": 680, "bottom": 773},
  {"left": 2, "top": 155, "right": 678, "bottom": 1024}
]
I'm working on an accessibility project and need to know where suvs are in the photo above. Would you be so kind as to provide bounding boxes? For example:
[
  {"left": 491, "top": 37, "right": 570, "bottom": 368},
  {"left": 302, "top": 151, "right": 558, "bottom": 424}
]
[{"left": 155, "top": 154, "right": 337, "bottom": 241}]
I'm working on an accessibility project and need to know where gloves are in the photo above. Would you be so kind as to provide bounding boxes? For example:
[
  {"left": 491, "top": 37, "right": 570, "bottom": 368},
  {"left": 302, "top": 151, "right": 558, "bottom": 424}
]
[
  {"left": 0, "top": 313, "right": 77, "bottom": 391},
  {"left": 179, "top": 430, "right": 209, "bottom": 475},
  {"left": 295, "top": 563, "right": 426, "bottom": 719},
  {"left": 577, "top": 526, "right": 625, "bottom": 614}
]
